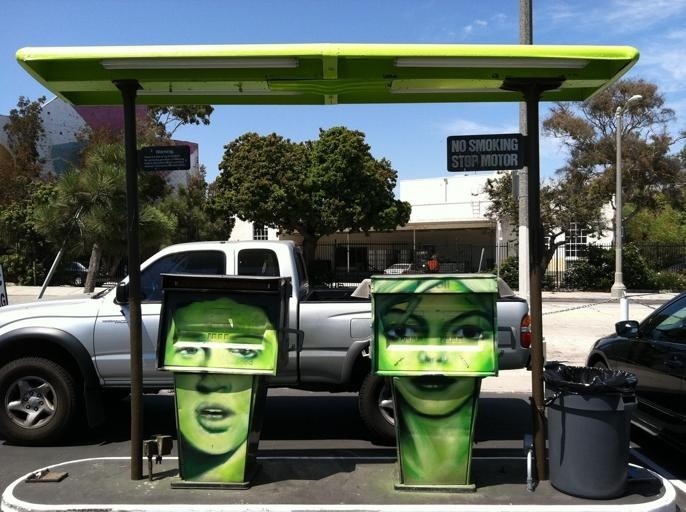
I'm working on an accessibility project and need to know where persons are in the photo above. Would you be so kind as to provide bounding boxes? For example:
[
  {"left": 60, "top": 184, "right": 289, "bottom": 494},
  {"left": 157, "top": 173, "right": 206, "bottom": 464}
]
[
  {"left": 164, "top": 295, "right": 286, "bottom": 482},
  {"left": 369, "top": 271, "right": 500, "bottom": 485}
]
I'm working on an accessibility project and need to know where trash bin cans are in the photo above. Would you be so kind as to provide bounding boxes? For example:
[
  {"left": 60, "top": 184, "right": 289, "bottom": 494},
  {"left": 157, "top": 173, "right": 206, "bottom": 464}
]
[{"left": 542, "top": 368, "right": 639, "bottom": 499}]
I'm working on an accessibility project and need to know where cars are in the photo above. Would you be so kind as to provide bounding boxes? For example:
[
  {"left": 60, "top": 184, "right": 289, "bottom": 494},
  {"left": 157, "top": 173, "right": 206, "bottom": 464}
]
[
  {"left": 586, "top": 291, "right": 686, "bottom": 492},
  {"left": 61, "top": 260, "right": 88, "bottom": 288},
  {"left": 382, "top": 262, "right": 426, "bottom": 275}
]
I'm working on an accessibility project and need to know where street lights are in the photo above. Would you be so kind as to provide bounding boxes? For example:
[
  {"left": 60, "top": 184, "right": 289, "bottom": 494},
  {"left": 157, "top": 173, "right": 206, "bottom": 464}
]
[{"left": 607, "top": 91, "right": 647, "bottom": 302}]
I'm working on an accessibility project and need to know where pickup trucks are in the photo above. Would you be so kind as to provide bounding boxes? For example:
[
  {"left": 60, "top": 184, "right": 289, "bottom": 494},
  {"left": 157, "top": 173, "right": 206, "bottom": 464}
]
[{"left": 0, "top": 236, "right": 535, "bottom": 448}]
